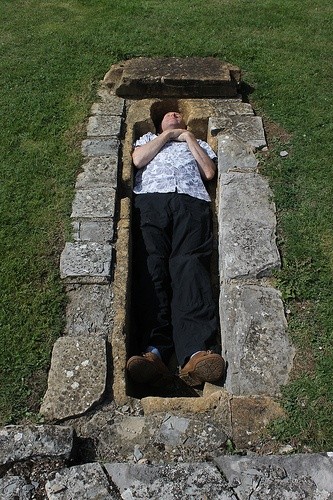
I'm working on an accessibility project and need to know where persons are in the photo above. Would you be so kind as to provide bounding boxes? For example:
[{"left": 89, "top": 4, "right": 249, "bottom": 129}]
[{"left": 124, "top": 111, "right": 226, "bottom": 391}]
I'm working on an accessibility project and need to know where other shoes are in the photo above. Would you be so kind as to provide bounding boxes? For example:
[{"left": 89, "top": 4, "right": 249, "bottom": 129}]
[
  {"left": 126, "top": 351, "right": 169, "bottom": 384},
  {"left": 178, "top": 350, "right": 225, "bottom": 386}
]
